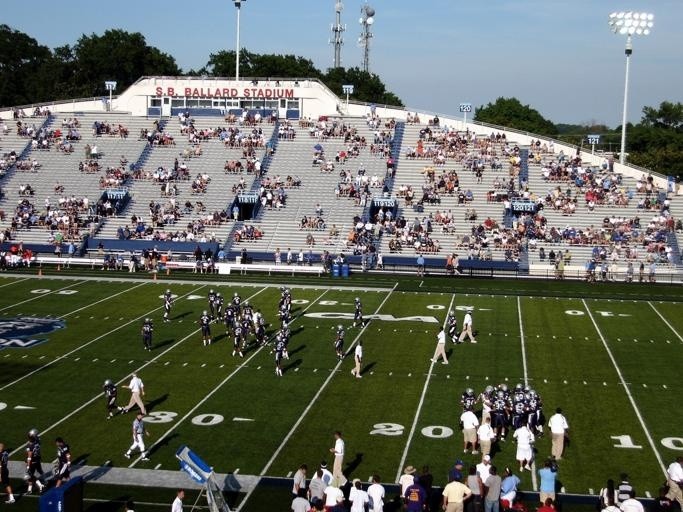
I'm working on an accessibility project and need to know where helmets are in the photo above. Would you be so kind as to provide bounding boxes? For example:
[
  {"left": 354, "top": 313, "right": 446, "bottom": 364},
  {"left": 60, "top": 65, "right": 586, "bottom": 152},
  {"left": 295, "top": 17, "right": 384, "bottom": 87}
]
[
  {"left": 29, "top": 429, "right": 38, "bottom": 437},
  {"left": 104, "top": 380, "right": 112, "bottom": 387},
  {"left": 466, "top": 383, "right": 536, "bottom": 402}
]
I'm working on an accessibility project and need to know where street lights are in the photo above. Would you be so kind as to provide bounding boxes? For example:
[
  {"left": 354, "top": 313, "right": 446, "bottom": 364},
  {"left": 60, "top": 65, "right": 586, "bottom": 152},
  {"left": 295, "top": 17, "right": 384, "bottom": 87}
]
[{"left": 608, "top": 10, "right": 654, "bottom": 162}]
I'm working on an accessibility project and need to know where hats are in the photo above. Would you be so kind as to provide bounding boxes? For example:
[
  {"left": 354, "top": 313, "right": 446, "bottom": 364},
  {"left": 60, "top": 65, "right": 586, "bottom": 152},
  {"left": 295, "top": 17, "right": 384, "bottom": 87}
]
[
  {"left": 403, "top": 465, "right": 417, "bottom": 474},
  {"left": 484, "top": 455, "right": 491, "bottom": 464},
  {"left": 455, "top": 459, "right": 465, "bottom": 466}
]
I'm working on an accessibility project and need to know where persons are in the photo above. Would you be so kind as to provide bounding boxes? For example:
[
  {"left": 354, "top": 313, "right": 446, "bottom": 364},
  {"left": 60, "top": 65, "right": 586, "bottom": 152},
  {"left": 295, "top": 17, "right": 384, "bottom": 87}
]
[
  {"left": 1, "top": 429, "right": 71, "bottom": 503},
  {"left": 399, "top": 455, "right": 557, "bottom": 511},
  {"left": 140, "top": 287, "right": 292, "bottom": 376},
  {"left": 0, "top": 109, "right": 682, "bottom": 282},
  {"left": 171, "top": 490, "right": 184, "bottom": 511},
  {"left": 292, "top": 431, "right": 385, "bottom": 511},
  {"left": 460, "top": 382, "right": 569, "bottom": 470},
  {"left": 599, "top": 456, "right": 683, "bottom": 512},
  {"left": 430, "top": 310, "right": 477, "bottom": 364},
  {"left": 335, "top": 297, "right": 366, "bottom": 378},
  {"left": 103, "top": 372, "right": 151, "bottom": 461}
]
